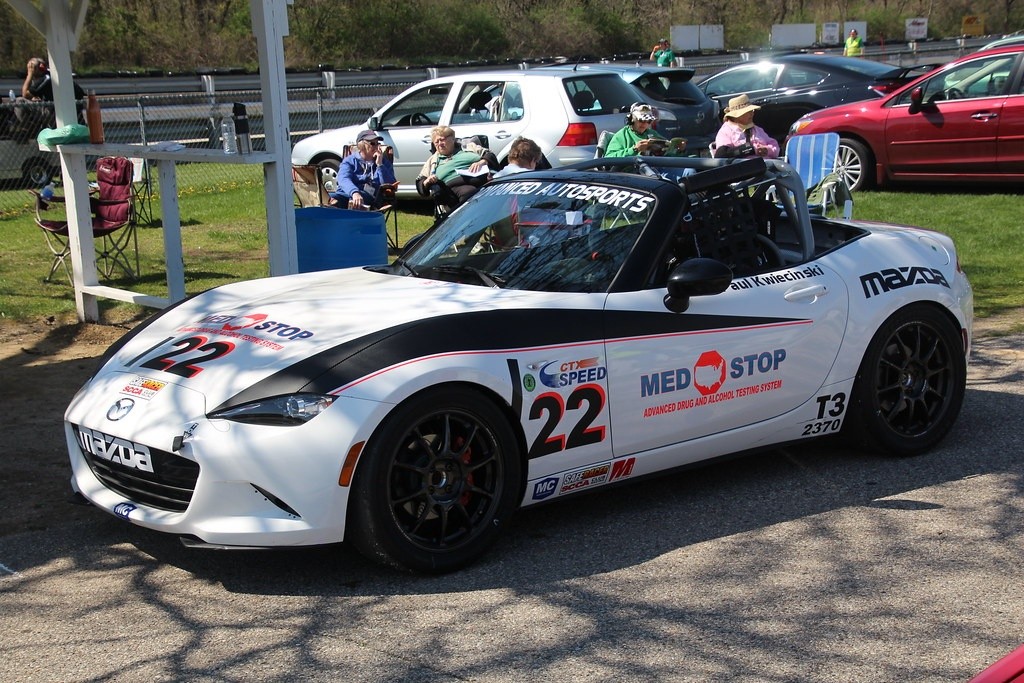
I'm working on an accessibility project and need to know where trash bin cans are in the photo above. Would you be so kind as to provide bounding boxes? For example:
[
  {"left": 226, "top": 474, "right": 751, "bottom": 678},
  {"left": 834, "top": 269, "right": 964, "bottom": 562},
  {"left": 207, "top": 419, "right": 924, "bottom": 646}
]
[{"left": 290, "top": 205, "right": 389, "bottom": 274}]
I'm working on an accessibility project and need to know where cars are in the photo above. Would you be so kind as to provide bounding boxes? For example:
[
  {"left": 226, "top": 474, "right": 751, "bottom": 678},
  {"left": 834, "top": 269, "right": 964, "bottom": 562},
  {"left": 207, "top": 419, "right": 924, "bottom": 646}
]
[
  {"left": 783, "top": 43, "right": 1024, "bottom": 195},
  {"left": 694, "top": 51, "right": 947, "bottom": 157},
  {"left": 0, "top": 88, "right": 67, "bottom": 190},
  {"left": 941, "top": 35, "right": 1024, "bottom": 97}
]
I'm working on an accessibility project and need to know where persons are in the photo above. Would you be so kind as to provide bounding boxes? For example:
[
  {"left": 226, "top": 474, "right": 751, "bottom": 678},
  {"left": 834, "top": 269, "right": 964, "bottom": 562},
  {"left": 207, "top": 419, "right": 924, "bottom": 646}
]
[
  {"left": 604, "top": 100, "right": 695, "bottom": 185},
  {"left": 415, "top": 126, "right": 498, "bottom": 208},
  {"left": 715, "top": 94, "right": 779, "bottom": 160},
  {"left": 21, "top": 59, "right": 84, "bottom": 124},
  {"left": 493, "top": 137, "right": 541, "bottom": 178},
  {"left": 329, "top": 129, "right": 398, "bottom": 213},
  {"left": 650, "top": 38, "right": 677, "bottom": 88},
  {"left": 843, "top": 29, "right": 865, "bottom": 60}
]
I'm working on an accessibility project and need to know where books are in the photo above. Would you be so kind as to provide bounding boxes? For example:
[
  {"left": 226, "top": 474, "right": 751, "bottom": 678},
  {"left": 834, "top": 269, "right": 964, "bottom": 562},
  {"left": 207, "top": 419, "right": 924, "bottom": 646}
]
[
  {"left": 347, "top": 200, "right": 370, "bottom": 211},
  {"left": 646, "top": 138, "right": 687, "bottom": 157}
]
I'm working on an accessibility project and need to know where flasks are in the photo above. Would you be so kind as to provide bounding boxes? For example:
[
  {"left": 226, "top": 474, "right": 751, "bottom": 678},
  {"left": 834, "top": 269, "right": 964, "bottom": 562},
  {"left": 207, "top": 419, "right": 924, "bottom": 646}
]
[{"left": 232, "top": 102, "right": 253, "bottom": 154}]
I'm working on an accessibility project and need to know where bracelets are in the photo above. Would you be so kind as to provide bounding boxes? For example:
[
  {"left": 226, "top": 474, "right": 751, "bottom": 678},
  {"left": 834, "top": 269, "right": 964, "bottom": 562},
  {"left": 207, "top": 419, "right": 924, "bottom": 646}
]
[{"left": 633, "top": 145, "right": 638, "bottom": 153}]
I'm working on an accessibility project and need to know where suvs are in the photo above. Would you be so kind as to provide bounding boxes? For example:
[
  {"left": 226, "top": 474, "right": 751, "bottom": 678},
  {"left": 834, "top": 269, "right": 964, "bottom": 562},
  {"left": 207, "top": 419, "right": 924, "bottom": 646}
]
[{"left": 288, "top": 57, "right": 718, "bottom": 208}]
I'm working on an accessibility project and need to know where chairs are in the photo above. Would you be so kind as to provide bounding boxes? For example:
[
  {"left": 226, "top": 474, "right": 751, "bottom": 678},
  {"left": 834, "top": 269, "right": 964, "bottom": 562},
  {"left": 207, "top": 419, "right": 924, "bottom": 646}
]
[
  {"left": 326, "top": 142, "right": 398, "bottom": 254},
  {"left": 464, "top": 91, "right": 493, "bottom": 123},
  {"left": 708, "top": 140, "right": 784, "bottom": 204},
  {"left": 595, "top": 131, "right": 698, "bottom": 229},
  {"left": 574, "top": 91, "right": 594, "bottom": 112},
  {"left": 766, "top": 132, "right": 855, "bottom": 220},
  {"left": 291, "top": 166, "right": 331, "bottom": 207},
  {"left": 429, "top": 134, "right": 493, "bottom": 260},
  {"left": 122, "top": 149, "right": 154, "bottom": 230},
  {"left": 24, "top": 155, "right": 142, "bottom": 292}
]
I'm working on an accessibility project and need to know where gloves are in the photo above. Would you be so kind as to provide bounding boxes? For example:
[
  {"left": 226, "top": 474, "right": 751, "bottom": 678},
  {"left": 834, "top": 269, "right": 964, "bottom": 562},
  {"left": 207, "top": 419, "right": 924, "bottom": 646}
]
[{"left": 653, "top": 46, "right": 660, "bottom": 53}]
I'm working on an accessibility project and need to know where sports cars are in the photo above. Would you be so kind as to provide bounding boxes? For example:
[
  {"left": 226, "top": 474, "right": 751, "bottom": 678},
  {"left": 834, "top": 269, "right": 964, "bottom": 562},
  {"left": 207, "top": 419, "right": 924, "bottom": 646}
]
[{"left": 59, "top": 160, "right": 978, "bottom": 583}]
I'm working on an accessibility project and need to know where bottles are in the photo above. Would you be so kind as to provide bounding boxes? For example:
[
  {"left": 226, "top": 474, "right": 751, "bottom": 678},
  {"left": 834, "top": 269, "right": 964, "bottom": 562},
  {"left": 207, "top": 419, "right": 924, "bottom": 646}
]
[
  {"left": 9, "top": 90, "right": 15, "bottom": 103},
  {"left": 221, "top": 112, "right": 237, "bottom": 154},
  {"left": 86, "top": 88, "right": 105, "bottom": 144},
  {"left": 40, "top": 182, "right": 55, "bottom": 200}
]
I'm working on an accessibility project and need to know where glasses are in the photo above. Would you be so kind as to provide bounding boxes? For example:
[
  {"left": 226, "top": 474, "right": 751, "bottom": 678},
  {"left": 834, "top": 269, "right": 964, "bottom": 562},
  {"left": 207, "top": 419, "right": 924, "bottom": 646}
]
[
  {"left": 365, "top": 141, "right": 381, "bottom": 146},
  {"left": 637, "top": 118, "right": 652, "bottom": 124}
]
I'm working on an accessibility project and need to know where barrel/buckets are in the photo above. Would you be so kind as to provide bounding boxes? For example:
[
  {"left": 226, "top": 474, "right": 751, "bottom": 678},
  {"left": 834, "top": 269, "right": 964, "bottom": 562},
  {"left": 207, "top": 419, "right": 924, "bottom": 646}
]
[{"left": 295, "top": 207, "right": 388, "bottom": 275}]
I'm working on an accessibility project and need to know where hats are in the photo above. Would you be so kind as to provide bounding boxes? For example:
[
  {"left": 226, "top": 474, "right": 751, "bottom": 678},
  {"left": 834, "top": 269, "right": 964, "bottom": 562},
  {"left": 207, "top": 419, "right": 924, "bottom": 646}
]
[
  {"left": 629, "top": 101, "right": 659, "bottom": 120},
  {"left": 849, "top": 29, "right": 858, "bottom": 36},
  {"left": 658, "top": 38, "right": 670, "bottom": 45},
  {"left": 356, "top": 130, "right": 383, "bottom": 142},
  {"left": 722, "top": 94, "right": 762, "bottom": 121}
]
